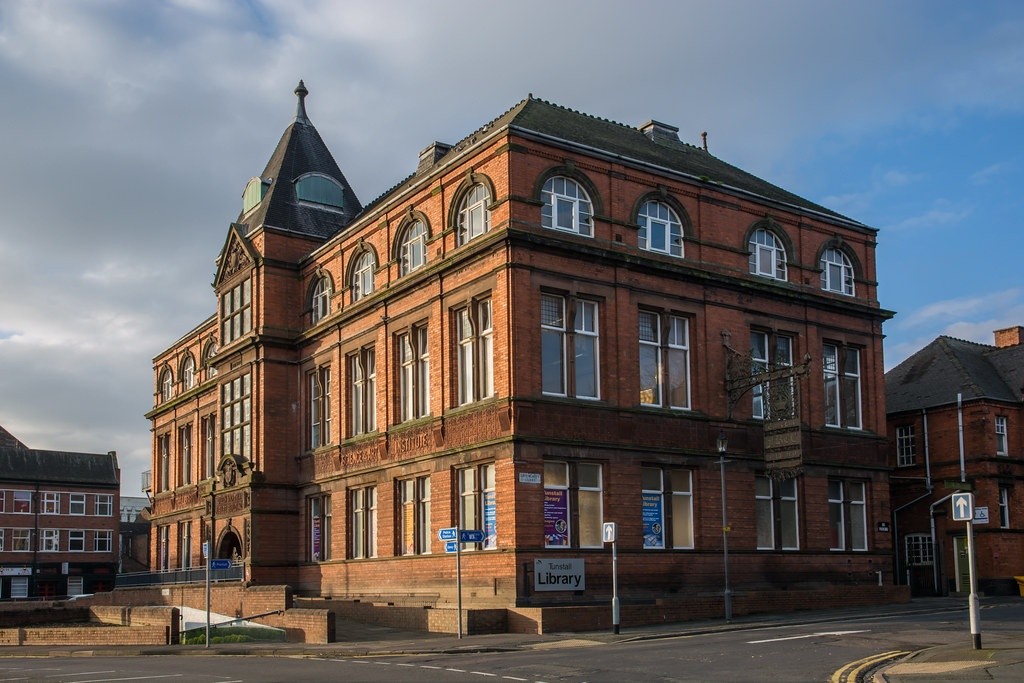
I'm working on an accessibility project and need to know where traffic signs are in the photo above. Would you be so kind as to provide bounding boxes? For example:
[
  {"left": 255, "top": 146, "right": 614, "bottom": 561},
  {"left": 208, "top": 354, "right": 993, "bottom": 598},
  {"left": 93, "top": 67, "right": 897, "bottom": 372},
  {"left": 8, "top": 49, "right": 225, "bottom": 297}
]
[{"left": 438, "top": 527, "right": 486, "bottom": 554}]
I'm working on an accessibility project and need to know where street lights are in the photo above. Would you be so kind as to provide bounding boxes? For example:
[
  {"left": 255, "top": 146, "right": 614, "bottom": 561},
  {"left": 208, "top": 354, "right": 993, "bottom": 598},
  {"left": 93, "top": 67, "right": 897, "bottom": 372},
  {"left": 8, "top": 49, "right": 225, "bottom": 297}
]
[{"left": 717, "top": 428, "right": 734, "bottom": 624}]
